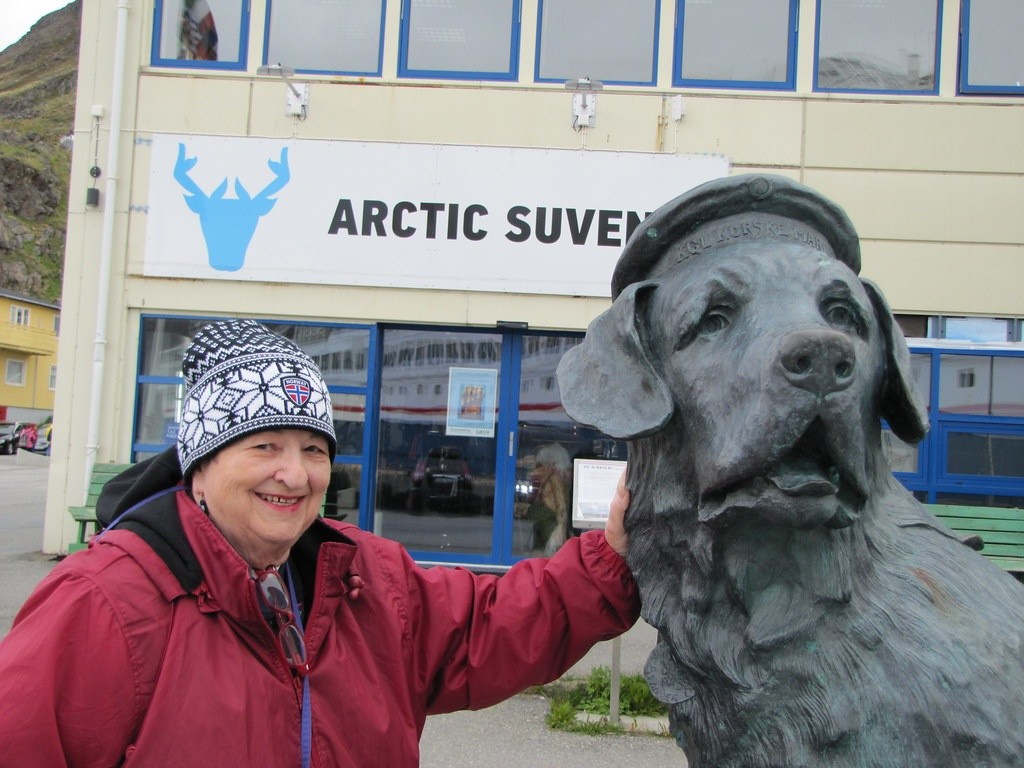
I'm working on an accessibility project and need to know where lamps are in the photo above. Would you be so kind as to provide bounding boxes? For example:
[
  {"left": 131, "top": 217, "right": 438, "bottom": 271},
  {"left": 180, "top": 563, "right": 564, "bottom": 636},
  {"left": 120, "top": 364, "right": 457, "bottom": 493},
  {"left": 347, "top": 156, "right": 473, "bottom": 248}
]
[
  {"left": 256, "top": 62, "right": 310, "bottom": 121},
  {"left": 563, "top": 77, "right": 603, "bottom": 132}
]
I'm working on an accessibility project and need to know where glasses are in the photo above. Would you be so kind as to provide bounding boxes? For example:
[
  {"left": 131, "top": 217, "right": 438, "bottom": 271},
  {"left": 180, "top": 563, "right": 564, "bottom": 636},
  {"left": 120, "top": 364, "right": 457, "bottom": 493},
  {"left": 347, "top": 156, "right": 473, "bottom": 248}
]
[{"left": 257, "top": 565, "right": 309, "bottom": 677}]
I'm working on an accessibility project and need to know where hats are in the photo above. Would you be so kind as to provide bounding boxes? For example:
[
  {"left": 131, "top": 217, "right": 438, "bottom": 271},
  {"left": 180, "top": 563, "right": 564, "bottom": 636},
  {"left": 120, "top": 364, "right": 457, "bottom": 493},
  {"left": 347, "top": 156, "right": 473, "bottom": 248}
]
[{"left": 177, "top": 319, "right": 337, "bottom": 484}]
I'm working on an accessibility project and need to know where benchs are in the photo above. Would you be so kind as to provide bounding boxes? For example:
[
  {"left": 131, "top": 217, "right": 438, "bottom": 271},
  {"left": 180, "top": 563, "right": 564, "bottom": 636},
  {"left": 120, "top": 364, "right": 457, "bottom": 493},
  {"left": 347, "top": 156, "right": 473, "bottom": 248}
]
[
  {"left": 65, "top": 463, "right": 327, "bottom": 556},
  {"left": 915, "top": 505, "right": 1024, "bottom": 575}
]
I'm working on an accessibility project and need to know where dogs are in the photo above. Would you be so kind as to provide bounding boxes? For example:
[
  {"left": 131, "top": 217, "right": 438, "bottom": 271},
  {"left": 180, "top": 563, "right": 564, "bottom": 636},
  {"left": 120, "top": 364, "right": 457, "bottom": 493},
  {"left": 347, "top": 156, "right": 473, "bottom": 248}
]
[{"left": 552, "top": 239, "right": 1024, "bottom": 768}]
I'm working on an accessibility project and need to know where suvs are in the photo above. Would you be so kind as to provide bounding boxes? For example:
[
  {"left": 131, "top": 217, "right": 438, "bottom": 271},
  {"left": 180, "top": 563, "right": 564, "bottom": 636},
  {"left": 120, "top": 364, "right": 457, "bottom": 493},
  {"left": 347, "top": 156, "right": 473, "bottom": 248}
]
[
  {"left": 407, "top": 446, "right": 490, "bottom": 517},
  {"left": 0, "top": 422, "right": 35, "bottom": 454}
]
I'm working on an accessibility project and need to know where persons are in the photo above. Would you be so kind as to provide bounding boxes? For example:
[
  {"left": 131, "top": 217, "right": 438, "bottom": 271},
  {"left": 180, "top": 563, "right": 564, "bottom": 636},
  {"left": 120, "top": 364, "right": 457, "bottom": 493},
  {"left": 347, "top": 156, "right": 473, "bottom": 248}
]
[
  {"left": 0, "top": 320, "right": 643, "bottom": 768},
  {"left": 521, "top": 443, "right": 572, "bottom": 560},
  {"left": 46, "top": 423, "right": 52, "bottom": 456},
  {"left": 19, "top": 424, "right": 37, "bottom": 452},
  {"left": 30, "top": 423, "right": 38, "bottom": 452}
]
